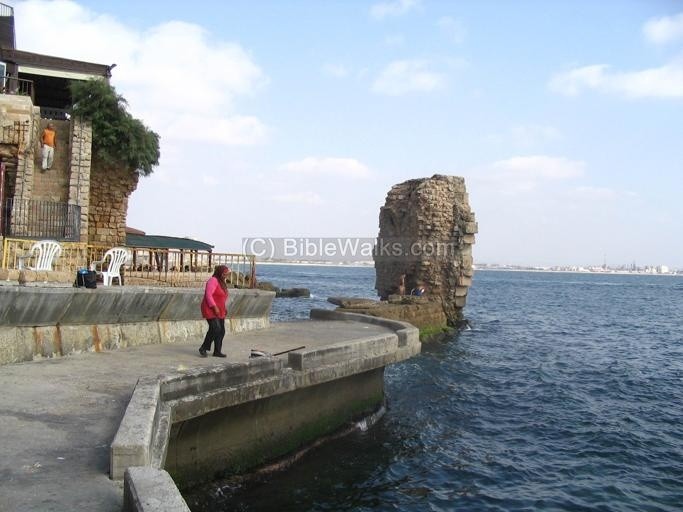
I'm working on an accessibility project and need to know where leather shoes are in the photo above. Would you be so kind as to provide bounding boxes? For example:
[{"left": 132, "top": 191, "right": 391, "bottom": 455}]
[
  {"left": 199, "top": 346, "right": 206, "bottom": 355},
  {"left": 213, "top": 352, "right": 226, "bottom": 357}
]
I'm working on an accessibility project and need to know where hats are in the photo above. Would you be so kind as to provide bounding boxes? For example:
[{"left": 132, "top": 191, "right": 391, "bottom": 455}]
[{"left": 223, "top": 269, "right": 229, "bottom": 273}]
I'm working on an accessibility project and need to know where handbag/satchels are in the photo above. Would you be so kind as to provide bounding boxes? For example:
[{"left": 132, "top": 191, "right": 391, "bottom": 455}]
[{"left": 73, "top": 269, "right": 96, "bottom": 289}]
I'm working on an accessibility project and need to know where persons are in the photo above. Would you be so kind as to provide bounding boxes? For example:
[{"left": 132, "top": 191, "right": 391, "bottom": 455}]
[
  {"left": 40, "top": 122, "right": 56, "bottom": 170},
  {"left": 199, "top": 265, "right": 231, "bottom": 357}
]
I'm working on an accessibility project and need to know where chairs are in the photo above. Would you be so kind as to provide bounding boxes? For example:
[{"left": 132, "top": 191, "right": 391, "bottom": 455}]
[
  {"left": 18, "top": 239, "right": 62, "bottom": 270},
  {"left": 90, "top": 247, "right": 132, "bottom": 287}
]
